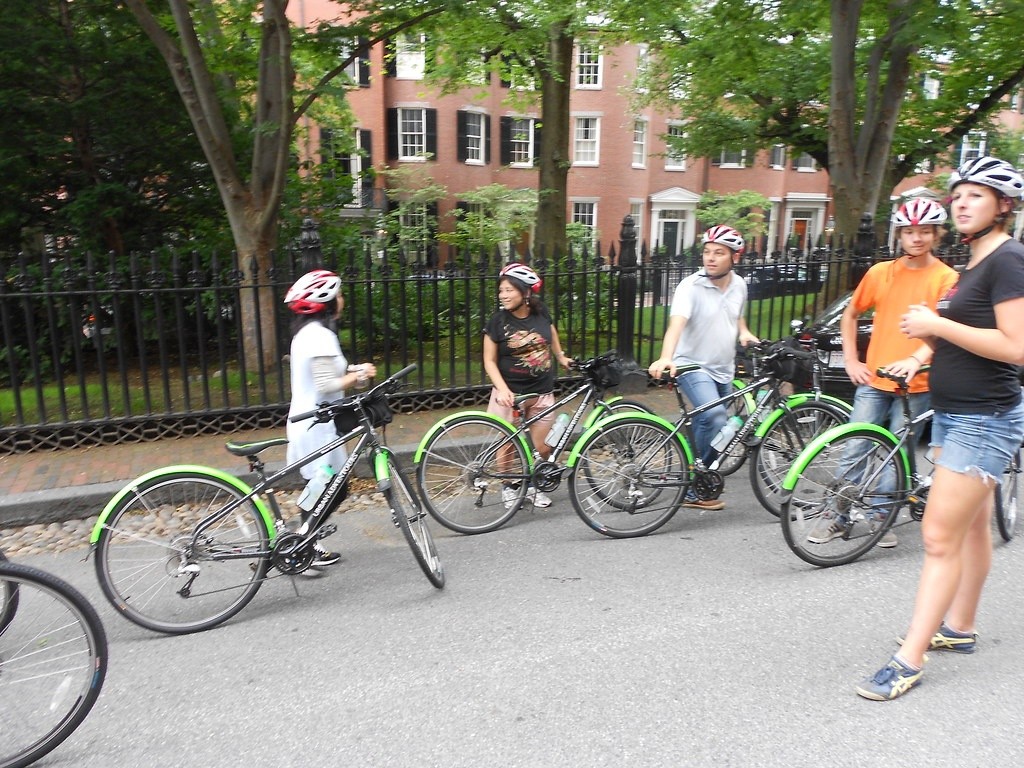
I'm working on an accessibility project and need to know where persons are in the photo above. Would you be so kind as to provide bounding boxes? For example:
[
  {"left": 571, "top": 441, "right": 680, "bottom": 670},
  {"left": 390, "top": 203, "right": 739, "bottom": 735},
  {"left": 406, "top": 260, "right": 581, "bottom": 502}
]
[
  {"left": 483, "top": 264, "right": 575, "bottom": 509},
  {"left": 853, "top": 157, "right": 1024, "bottom": 701},
  {"left": 284, "top": 270, "right": 377, "bottom": 577},
  {"left": 648, "top": 225, "right": 760, "bottom": 510},
  {"left": 807, "top": 199, "right": 960, "bottom": 548}
]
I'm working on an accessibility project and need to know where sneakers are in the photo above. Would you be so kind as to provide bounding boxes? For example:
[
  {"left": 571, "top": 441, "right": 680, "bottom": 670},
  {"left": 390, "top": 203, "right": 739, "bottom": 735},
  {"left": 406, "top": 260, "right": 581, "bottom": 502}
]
[
  {"left": 299, "top": 561, "right": 326, "bottom": 579},
  {"left": 892, "top": 620, "right": 983, "bottom": 654},
  {"left": 523, "top": 485, "right": 553, "bottom": 508},
  {"left": 502, "top": 486, "right": 525, "bottom": 511},
  {"left": 303, "top": 542, "right": 341, "bottom": 567},
  {"left": 868, "top": 520, "right": 898, "bottom": 549},
  {"left": 680, "top": 495, "right": 727, "bottom": 510},
  {"left": 854, "top": 653, "right": 924, "bottom": 702},
  {"left": 805, "top": 521, "right": 846, "bottom": 545}
]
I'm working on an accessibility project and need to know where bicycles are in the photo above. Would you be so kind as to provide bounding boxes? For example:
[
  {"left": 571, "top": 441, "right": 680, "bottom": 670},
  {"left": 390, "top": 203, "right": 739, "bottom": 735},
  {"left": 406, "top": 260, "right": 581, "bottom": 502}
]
[
  {"left": 774, "top": 364, "right": 1024, "bottom": 569},
  {"left": 80, "top": 362, "right": 447, "bottom": 636},
  {"left": 0, "top": 555, "right": 110, "bottom": 768},
  {"left": 559, "top": 315, "right": 880, "bottom": 539},
  {"left": 413, "top": 349, "right": 672, "bottom": 536}
]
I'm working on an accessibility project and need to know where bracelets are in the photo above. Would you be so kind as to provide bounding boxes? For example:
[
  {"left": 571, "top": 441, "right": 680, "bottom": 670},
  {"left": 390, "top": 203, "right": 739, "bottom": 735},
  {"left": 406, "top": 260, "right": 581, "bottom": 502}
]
[
  {"left": 353, "top": 370, "right": 370, "bottom": 389},
  {"left": 910, "top": 355, "right": 922, "bottom": 368},
  {"left": 555, "top": 351, "right": 565, "bottom": 358}
]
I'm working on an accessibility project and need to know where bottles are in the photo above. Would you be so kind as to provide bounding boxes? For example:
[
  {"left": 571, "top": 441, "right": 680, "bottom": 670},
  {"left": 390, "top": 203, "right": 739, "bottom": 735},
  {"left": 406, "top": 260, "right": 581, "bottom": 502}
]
[
  {"left": 756, "top": 388, "right": 771, "bottom": 423},
  {"left": 710, "top": 416, "right": 744, "bottom": 452},
  {"left": 297, "top": 463, "right": 335, "bottom": 511},
  {"left": 544, "top": 413, "right": 571, "bottom": 447}
]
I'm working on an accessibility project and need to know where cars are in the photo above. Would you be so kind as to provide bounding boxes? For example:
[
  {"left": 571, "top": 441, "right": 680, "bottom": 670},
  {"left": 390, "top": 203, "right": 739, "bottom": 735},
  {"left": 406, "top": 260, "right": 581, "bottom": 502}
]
[{"left": 786, "top": 280, "right": 960, "bottom": 444}]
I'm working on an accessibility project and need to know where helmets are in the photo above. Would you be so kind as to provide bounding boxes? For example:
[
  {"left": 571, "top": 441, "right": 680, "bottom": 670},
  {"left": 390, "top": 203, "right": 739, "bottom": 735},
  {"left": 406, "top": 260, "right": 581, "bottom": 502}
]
[
  {"left": 500, "top": 263, "right": 543, "bottom": 295},
  {"left": 700, "top": 223, "right": 747, "bottom": 257},
  {"left": 946, "top": 156, "right": 1024, "bottom": 199},
  {"left": 889, "top": 198, "right": 949, "bottom": 228},
  {"left": 283, "top": 269, "right": 341, "bottom": 316}
]
